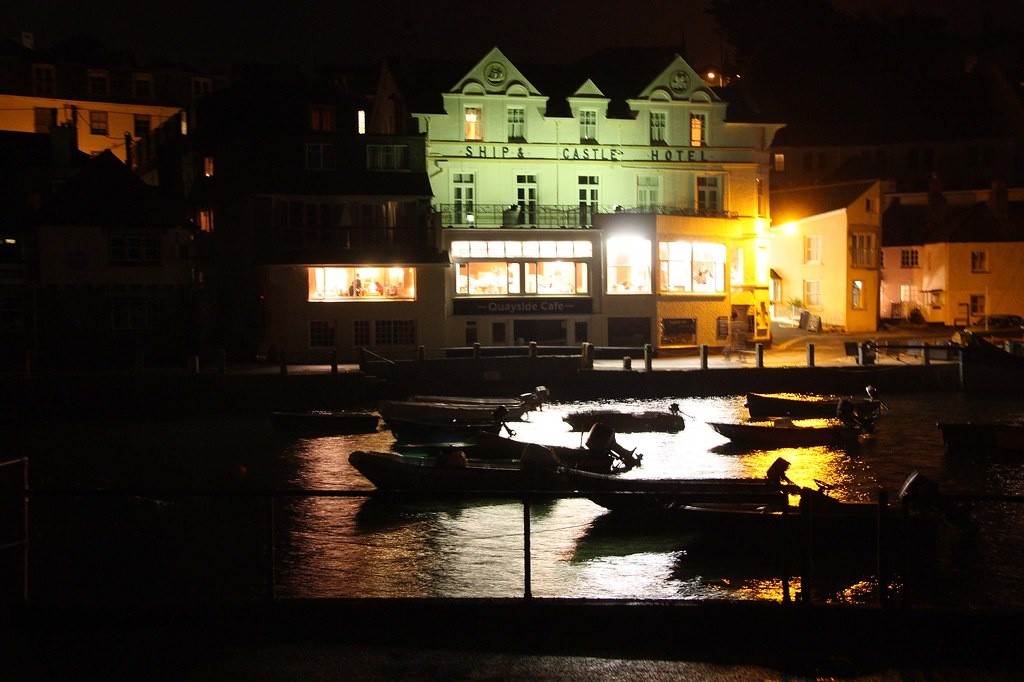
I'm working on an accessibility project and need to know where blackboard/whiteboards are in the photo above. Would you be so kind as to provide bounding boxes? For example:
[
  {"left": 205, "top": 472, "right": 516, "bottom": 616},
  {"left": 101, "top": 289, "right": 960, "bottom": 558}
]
[
  {"left": 891, "top": 304, "right": 901, "bottom": 316},
  {"left": 800, "top": 312, "right": 811, "bottom": 329},
  {"left": 807, "top": 316, "right": 822, "bottom": 331}
]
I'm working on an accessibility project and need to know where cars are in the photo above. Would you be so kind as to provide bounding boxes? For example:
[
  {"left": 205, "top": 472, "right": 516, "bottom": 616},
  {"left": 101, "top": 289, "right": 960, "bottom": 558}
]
[{"left": 963, "top": 316, "right": 1024, "bottom": 338}]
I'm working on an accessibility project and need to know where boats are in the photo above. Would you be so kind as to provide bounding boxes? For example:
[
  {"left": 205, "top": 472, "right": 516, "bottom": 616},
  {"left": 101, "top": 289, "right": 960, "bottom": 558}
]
[
  {"left": 711, "top": 400, "right": 864, "bottom": 447},
  {"left": 268, "top": 411, "right": 381, "bottom": 438},
  {"left": 348, "top": 386, "right": 685, "bottom": 501},
  {"left": 747, "top": 384, "right": 884, "bottom": 421},
  {"left": 935, "top": 421, "right": 1024, "bottom": 461},
  {"left": 556, "top": 458, "right": 789, "bottom": 511},
  {"left": 967, "top": 330, "right": 1024, "bottom": 401},
  {"left": 662, "top": 471, "right": 938, "bottom": 547}
]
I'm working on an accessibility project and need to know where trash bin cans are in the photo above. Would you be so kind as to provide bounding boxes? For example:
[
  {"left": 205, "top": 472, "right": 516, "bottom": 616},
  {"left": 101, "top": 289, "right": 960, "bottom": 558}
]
[{"left": 844, "top": 342, "right": 859, "bottom": 355}]
